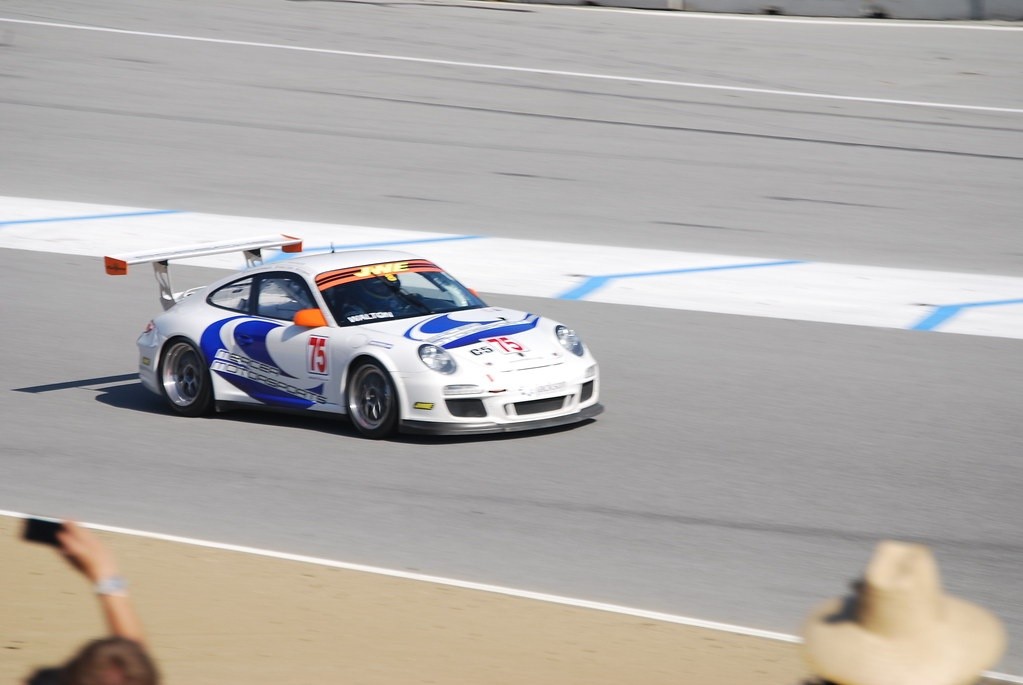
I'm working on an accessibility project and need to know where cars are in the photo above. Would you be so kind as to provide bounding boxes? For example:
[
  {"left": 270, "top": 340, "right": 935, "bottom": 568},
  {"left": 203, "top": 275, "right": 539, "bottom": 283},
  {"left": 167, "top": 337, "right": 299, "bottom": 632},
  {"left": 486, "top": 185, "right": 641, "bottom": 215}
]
[{"left": 101, "top": 230, "right": 607, "bottom": 439}]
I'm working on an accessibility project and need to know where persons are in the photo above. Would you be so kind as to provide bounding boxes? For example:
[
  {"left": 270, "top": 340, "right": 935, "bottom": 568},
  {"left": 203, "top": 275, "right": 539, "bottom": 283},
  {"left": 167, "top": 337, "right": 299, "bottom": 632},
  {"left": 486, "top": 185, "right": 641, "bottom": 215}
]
[{"left": 355, "top": 274, "right": 417, "bottom": 317}]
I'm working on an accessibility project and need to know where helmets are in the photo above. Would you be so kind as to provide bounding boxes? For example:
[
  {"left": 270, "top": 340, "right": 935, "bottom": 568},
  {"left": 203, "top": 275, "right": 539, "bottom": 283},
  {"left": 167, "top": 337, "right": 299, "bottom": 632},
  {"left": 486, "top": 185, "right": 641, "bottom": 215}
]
[{"left": 357, "top": 275, "right": 401, "bottom": 307}]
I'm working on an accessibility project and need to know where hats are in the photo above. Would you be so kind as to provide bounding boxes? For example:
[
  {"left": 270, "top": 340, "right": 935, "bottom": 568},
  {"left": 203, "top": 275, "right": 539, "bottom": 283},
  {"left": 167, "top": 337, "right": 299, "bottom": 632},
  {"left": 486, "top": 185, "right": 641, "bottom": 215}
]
[{"left": 807, "top": 541, "right": 1004, "bottom": 684}]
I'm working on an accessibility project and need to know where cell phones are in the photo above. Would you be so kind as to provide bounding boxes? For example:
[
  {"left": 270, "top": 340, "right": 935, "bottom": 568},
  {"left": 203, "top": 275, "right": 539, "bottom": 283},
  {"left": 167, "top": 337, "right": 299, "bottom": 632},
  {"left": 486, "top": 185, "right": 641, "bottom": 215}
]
[{"left": 20, "top": 518, "right": 68, "bottom": 549}]
